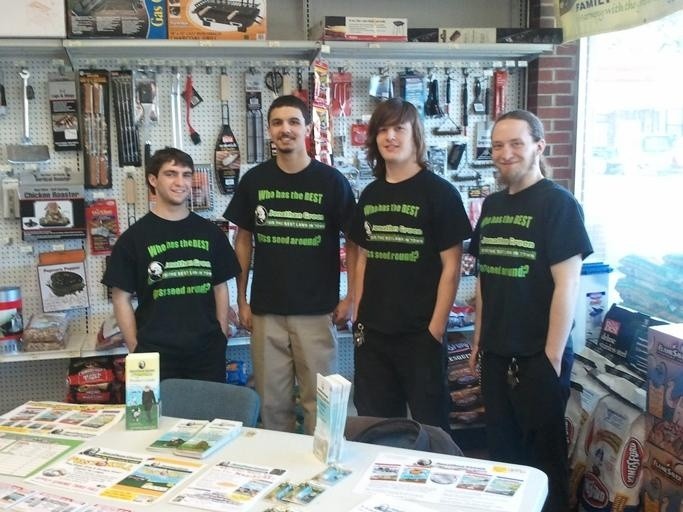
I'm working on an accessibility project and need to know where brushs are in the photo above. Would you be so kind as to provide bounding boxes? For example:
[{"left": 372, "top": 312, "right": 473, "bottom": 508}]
[{"left": 472, "top": 78, "right": 484, "bottom": 115}]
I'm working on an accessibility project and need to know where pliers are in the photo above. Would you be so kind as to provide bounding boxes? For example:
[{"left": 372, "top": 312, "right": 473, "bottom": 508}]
[{"left": 336, "top": 83, "right": 351, "bottom": 120}]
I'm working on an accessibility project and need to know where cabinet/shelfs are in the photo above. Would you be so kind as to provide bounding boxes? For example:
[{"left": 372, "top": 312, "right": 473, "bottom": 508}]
[
  {"left": 0, "top": 32, "right": 87, "bottom": 366},
  {"left": 62, "top": 36, "right": 321, "bottom": 358},
  {"left": 310, "top": 40, "right": 556, "bottom": 342}
]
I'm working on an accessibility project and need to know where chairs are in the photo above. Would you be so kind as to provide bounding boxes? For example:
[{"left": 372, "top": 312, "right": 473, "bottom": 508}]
[{"left": 160, "top": 378, "right": 261, "bottom": 429}]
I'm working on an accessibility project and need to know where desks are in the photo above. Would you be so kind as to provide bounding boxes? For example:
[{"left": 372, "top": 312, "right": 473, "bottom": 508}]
[{"left": 0, "top": 399, "right": 548, "bottom": 512}]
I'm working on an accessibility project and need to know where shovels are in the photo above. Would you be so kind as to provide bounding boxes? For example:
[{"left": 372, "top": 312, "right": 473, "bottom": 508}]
[{"left": 5, "top": 70, "right": 51, "bottom": 166}]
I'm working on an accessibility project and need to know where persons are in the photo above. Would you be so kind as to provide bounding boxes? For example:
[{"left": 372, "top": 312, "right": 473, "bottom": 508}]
[
  {"left": 220, "top": 95, "right": 357, "bottom": 434},
  {"left": 345, "top": 97, "right": 473, "bottom": 429},
  {"left": 99, "top": 148, "right": 241, "bottom": 382},
  {"left": 468, "top": 109, "right": 595, "bottom": 501}
]
